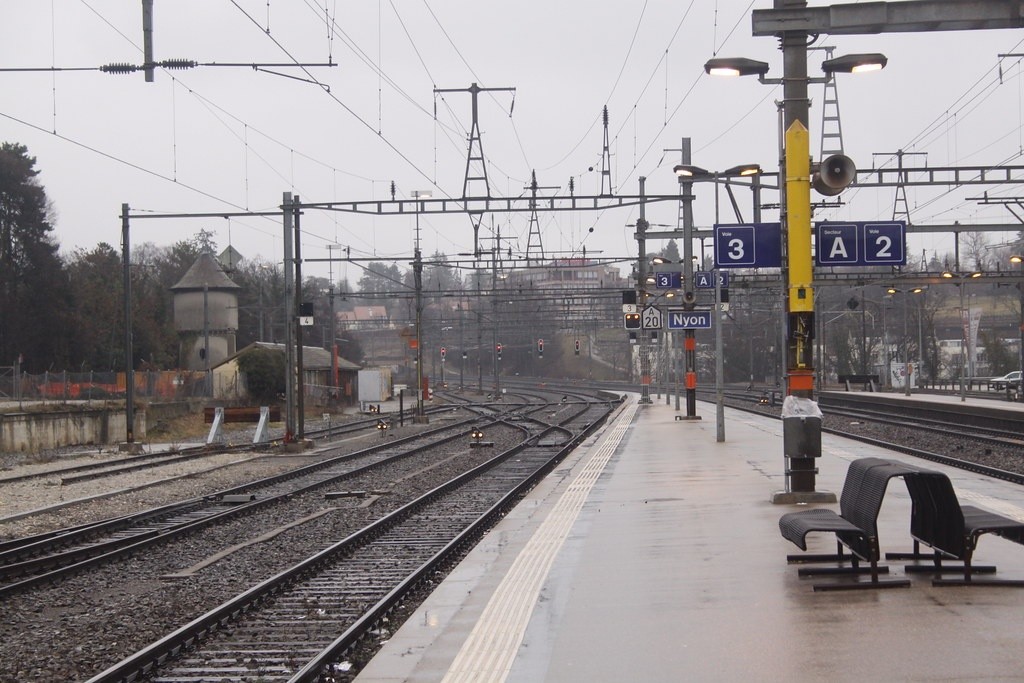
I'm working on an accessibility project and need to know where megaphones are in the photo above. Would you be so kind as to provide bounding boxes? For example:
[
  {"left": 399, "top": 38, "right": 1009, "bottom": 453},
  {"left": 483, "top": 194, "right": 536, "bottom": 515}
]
[
  {"left": 684, "top": 291, "right": 696, "bottom": 304},
  {"left": 812, "top": 155, "right": 857, "bottom": 196}
]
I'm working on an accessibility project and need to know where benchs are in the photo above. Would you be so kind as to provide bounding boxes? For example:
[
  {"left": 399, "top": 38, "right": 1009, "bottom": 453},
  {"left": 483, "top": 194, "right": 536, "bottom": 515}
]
[{"left": 779, "top": 458, "right": 1024, "bottom": 591}]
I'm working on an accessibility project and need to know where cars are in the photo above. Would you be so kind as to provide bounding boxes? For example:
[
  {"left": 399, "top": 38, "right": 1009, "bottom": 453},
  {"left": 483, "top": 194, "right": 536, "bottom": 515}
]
[{"left": 989, "top": 371, "right": 1023, "bottom": 388}]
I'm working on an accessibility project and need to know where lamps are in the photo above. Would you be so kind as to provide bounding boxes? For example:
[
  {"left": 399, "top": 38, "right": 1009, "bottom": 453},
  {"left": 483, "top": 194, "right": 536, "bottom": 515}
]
[
  {"left": 472, "top": 429, "right": 483, "bottom": 438},
  {"left": 378, "top": 423, "right": 387, "bottom": 429}
]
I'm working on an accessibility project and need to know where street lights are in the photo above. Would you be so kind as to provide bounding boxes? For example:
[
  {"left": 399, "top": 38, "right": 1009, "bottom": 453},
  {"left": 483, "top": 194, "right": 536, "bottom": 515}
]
[
  {"left": 652, "top": 255, "right": 698, "bottom": 410},
  {"left": 644, "top": 293, "right": 674, "bottom": 399},
  {"left": 885, "top": 287, "right": 923, "bottom": 397},
  {"left": 941, "top": 269, "right": 983, "bottom": 401},
  {"left": 704, "top": 52, "right": 889, "bottom": 508},
  {"left": 673, "top": 163, "right": 760, "bottom": 442}
]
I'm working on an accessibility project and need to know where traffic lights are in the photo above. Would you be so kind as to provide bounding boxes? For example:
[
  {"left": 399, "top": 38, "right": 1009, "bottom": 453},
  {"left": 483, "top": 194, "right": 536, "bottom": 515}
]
[
  {"left": 538, "top": 339, "right": 543, "bottom": 353},
  {"left": 574, "top": 340, "right": 581, "bottom": 351},
  {"left": 440, "top": 347, "right": 446, "bottom": 358},
  {"left": 496, "top": 343, "right": 501, "bottom": 354},
  {"left": 623, "top": 312, "right": 641, "bottom": 331}
]
